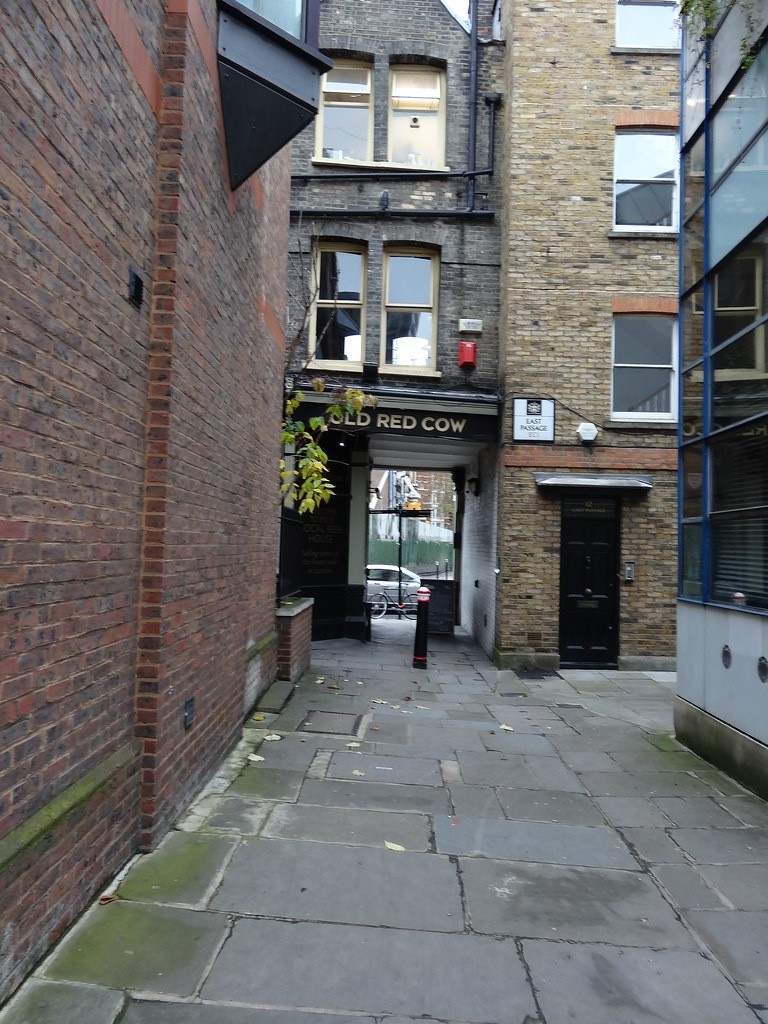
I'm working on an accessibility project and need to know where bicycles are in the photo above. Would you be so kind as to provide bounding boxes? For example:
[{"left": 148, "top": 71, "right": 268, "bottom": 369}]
[{"left": 366, "top": 582, "right": 419, "bottom": 620}]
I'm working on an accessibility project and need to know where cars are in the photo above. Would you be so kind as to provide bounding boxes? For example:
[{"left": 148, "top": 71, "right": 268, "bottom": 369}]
[{"left": 363, "top": 564, "right": 423, "bottom": 613}]
